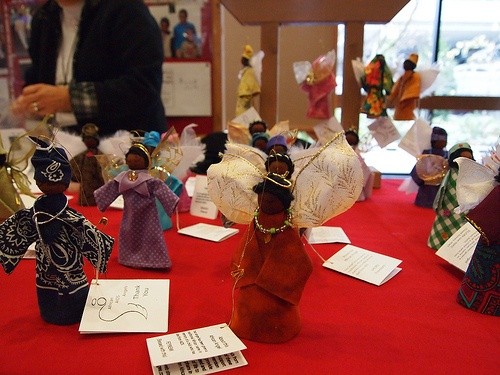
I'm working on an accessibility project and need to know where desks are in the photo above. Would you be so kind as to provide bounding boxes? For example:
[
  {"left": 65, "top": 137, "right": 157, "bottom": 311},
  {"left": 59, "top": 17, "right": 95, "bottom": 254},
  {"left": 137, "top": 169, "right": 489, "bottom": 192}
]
[{"left": 0, "top": 178, "right": 500, "bottom": 375}]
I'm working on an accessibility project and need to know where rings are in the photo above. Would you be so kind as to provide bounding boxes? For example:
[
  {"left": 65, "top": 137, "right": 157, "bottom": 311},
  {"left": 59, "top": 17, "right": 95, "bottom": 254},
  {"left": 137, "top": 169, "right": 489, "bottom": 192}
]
[{"left": 33, "top": 102, "right": 39, "bottom": 111}]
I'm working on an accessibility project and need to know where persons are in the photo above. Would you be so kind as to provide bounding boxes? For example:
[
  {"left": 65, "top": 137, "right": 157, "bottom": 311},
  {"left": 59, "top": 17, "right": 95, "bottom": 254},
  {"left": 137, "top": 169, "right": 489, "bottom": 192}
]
[
  {"left": 0, "top": 120, "right": 370, "bottom": 346},
  {"left": 176, "top": 28, "right": 203, "bottom": 58},
  {"left": 159, "top": 18, "right": 175, "bottom": 59},
  {"left": 410, "top": 127, "right": 500, "bottom": 317},
  {"left": 172, "top": 9, "right": 196, "bottom": 50},
  {"left": 234, "top": 44, "right": 260, "bottom": 116},
  {"left": 360, "top": 53, "right": 394, "bottom": 117},
  {"left": 10, "top": 0, "right": 167, "bottom": 137},
  {"left": 301, "top": 55, "right": 337, "bottom": 119},
  {"left": 385, "top": 53, "right": 421, "bottom": 120}
]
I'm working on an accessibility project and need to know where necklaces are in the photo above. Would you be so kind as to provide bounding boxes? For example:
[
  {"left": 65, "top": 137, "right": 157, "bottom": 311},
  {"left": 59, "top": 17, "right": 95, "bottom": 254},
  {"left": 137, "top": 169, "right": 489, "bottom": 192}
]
[
  {"left": 253, "top": 206, "right": 292, "bottom": 234},
  {"left": 60, "top": 33, "right": 76, "bottom": 84}
]
